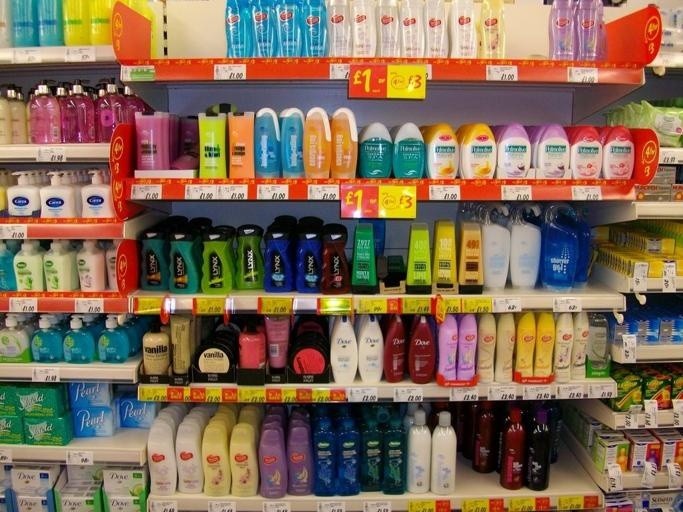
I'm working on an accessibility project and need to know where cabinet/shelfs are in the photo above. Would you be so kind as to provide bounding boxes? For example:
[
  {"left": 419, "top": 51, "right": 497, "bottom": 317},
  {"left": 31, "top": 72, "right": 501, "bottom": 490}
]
[{"left": 1, "top": 2, "right": 683, "bottom": 512}]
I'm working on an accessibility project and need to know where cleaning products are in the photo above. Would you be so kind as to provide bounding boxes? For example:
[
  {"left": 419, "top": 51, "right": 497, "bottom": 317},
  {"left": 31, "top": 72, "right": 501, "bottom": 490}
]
[
  {"left": 461, "top": 401, "right": 563, "bottom": 490},
  {"left": 313, "top": 402, "right": 460, "bottom": 494}
]
